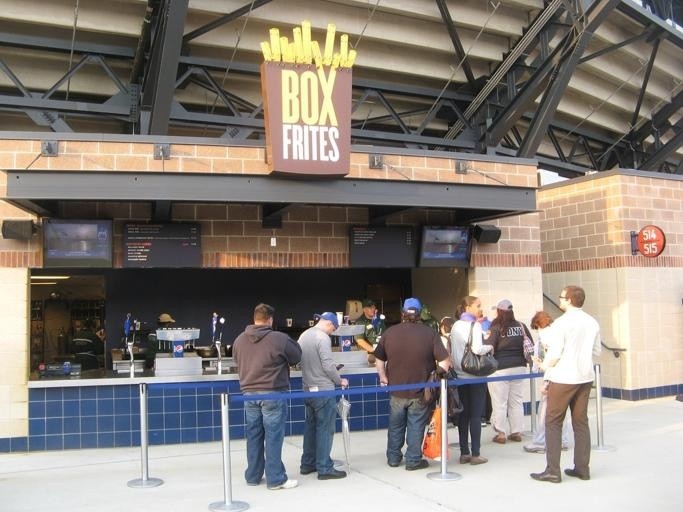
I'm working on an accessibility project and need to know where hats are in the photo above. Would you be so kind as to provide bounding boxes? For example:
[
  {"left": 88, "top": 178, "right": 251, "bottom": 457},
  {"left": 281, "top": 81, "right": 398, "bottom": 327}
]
[
  {"left": 491, "top": 299, "right": 513, "bottom": 312},
  {"left": 403, "top": 297, "right": 422, "bottom": 314},
  {"left": 313, "top": 311, "right": 339, "bottom": 330},
  {"left": 362, "top": 298, "right": 378, "bottom": 307},
  {"left": 158, "top": 313, "right": 177, "bottom": 324}
]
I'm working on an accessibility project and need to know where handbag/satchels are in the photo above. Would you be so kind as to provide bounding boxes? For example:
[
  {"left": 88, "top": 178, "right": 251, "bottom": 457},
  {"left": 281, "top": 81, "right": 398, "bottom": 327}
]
[{"left": 422, "top": 393, "right": 449, "bottom": 461}]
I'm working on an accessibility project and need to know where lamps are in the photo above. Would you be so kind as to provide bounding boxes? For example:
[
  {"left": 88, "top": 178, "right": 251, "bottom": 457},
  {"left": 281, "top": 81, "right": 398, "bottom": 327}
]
[{"left": 269, "top": 228, "right": 279, "bottom": 246}]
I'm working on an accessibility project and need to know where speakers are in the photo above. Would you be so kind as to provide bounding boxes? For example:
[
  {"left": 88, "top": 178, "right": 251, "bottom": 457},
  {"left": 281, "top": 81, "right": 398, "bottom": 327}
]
[
  {"left": 474, "top": 224, "right": 501, "bottom": 243},
  {"left": 3, "top": 220, "right": 33, "bottom": 239}
]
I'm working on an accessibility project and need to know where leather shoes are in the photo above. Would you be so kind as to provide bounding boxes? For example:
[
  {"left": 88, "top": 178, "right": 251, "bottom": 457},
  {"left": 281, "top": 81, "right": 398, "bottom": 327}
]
[
  {"left": 387, "top": 459, "right": 400, "bottom": 467},
  {"left": 529, "top": 469, "right": 561, "bottom": 483},
  {"left": 507, "top": 434, "right": 522, "bottom": 442},
  {"left": 522, "top": 442, "right": 545, "bottom": 455},
  {"left": 300, "top": 467, "right": 317, "bottom": 474},
  {"left": 470, "top": 456, "right": 488, "bottom": 464},
  {"left": 563, "top": 468, "right": 590, "bottom": 480},
  {"left": 318, "top": 470, "right": 346, "bottom": 480},
  {"left": 493, "top": 435, "right": 506, "bottom": 444},
  {"left": 406, "top": 459, "right": 429, "bottom": 471},
  {"left": 459, "top": 455, "right": 472, "bottom": 463}
]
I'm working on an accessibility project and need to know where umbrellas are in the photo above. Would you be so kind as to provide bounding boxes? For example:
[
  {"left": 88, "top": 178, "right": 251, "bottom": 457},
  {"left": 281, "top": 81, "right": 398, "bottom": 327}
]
[{"left": 336, "top": 384, "right": 351, "bottom": 475}]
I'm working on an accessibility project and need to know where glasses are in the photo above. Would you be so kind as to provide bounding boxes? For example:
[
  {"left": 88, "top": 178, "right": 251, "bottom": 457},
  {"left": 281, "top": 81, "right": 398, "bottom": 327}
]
[{"left": 557, "top": 295, "right": 568, "bottom": 300}]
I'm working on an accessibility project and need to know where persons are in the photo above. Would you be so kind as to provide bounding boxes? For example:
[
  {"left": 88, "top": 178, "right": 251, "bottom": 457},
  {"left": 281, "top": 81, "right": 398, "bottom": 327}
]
[
  {"left": 371, "top": 299, "right": 451, "bottom": 471},
  {"left": 296, "top": 311, "right": 348, "bottom": 480},
  {"left": 523, "top": 311, "right": 569, "bottom": 453},
  {"left": 146, "top": 313, "right": 174, "bottom": 370},
  {"left": 436, "top": 313, "right": 455, "bottom": 353},
  {"left": 419, "top": 305, "right": 438, "bottom": 332},
  {"left": 232, "top": 304, "right": 302, "bottom": 490},
  {"left": 530, "top": 286, "right": 600, "bottom": 483},
  {"left": 487, "top": 300, "right": 534, "bottom": 444},
  {"left": 451, "top": 296, "right": 493, "bottom": 464},
  {"left": 353, "top": 299, "right": 386, "bottom": 353},
  {"left": 473, "top": 310, "right": 493, "bottom": 427}
]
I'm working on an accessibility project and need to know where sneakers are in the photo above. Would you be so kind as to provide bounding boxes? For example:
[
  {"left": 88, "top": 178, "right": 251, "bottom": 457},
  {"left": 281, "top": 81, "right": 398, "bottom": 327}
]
[
  {"left": 561, "top": 445, "right": 570, "bottom": 453},
  {"left": 267, "top": 479, "right": 298, "bottom": 491}
]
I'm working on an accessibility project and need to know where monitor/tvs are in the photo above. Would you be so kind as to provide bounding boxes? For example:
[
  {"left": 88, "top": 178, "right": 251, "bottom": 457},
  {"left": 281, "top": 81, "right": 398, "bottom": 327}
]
[
  {"left": 418, "top": 224, "right": 469, "bottom": 269},
  {"left": 43, "top": 216, "right": 115, "bottom": 270}
]
